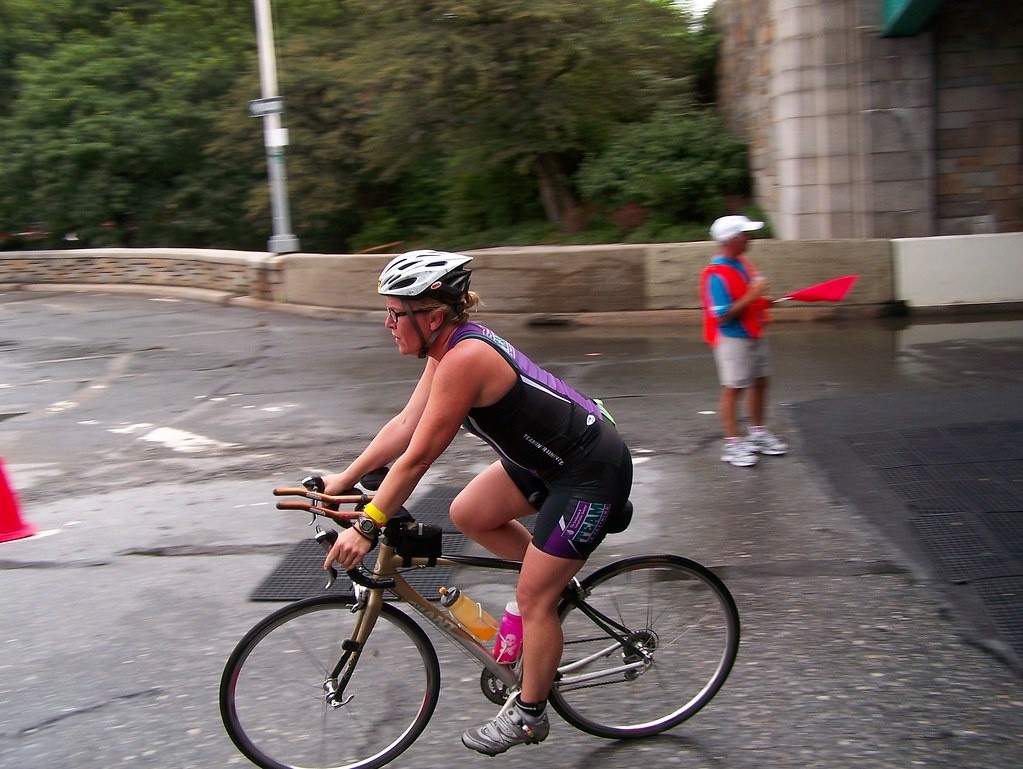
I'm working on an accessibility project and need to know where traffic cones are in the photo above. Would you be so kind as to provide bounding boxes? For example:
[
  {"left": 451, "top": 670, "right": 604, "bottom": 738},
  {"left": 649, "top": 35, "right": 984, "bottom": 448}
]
[{"left": 0, "top": 454, "right": 38, "bottom": 544}]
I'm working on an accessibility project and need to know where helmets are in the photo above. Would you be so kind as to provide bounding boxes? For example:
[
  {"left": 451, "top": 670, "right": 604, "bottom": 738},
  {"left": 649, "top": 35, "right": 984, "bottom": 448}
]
[{"left": 377, "top": 249, "right": 474, "bottom": 309}]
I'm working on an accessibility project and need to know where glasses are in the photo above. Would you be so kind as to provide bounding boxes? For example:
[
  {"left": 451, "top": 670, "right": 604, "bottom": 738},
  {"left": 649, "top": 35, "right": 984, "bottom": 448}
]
[{"left": 386, "top": 307, "right": 434, "bottom": 322}]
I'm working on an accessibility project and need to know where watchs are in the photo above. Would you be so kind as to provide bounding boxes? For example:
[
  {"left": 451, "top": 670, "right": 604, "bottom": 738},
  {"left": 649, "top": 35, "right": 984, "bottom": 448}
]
[{"left": 357, "top": 515, "right": 382, "bottom": 536}]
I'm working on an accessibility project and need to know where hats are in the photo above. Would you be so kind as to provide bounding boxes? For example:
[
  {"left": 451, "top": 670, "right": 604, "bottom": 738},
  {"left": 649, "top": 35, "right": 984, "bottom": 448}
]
[{"left": 709, "top": 215, "right": 764, "bottom": 242}]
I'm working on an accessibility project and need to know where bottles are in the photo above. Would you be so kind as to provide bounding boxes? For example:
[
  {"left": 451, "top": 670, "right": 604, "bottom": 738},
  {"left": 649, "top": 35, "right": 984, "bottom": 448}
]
[
  {"left": 492, "top": 601, "right": 523, "bottom": 663},
  {"left": 439, "top": 587, "right": 498, "bottom": 641},
  {"left": 751, "top": 272, "right": 763, "bottom": 284}
]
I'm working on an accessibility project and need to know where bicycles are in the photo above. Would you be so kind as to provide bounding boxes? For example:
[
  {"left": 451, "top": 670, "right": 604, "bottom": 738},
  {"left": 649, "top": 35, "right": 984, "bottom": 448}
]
[{"left": 218, "top": 464, "right": 741, "bottom": 769}]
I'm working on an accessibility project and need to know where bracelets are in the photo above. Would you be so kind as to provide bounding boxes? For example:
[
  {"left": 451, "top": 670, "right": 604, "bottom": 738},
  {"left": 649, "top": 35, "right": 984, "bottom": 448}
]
[{"left": 364, "top": 504, "right": 388, "bottom": 525}]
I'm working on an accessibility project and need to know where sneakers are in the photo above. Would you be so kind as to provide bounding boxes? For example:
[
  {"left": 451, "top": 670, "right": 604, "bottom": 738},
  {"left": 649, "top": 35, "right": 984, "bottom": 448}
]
[
  {"left": 461, "top": 705, "right": 550, "bottom": 754},
  {"left": 720, "top": 440, "right": 759, "bottom": 467},
  {"left": 745, "top": 432, "right": 788, "bottom": 455}
]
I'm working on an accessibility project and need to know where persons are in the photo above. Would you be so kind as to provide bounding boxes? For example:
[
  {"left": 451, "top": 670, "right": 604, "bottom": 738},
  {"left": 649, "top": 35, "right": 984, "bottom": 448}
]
[
  {"left": 700, "top": 215, "right": 790, "bottom": 466},
  {"left": 320, "top": 249, "right": 634, "bottom": 753}
]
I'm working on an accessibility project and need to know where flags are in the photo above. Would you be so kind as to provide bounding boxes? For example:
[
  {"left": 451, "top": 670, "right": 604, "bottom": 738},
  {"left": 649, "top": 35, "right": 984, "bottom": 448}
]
[{"left": 784, "top": 274, "right": 859, "bottom": 302}]
